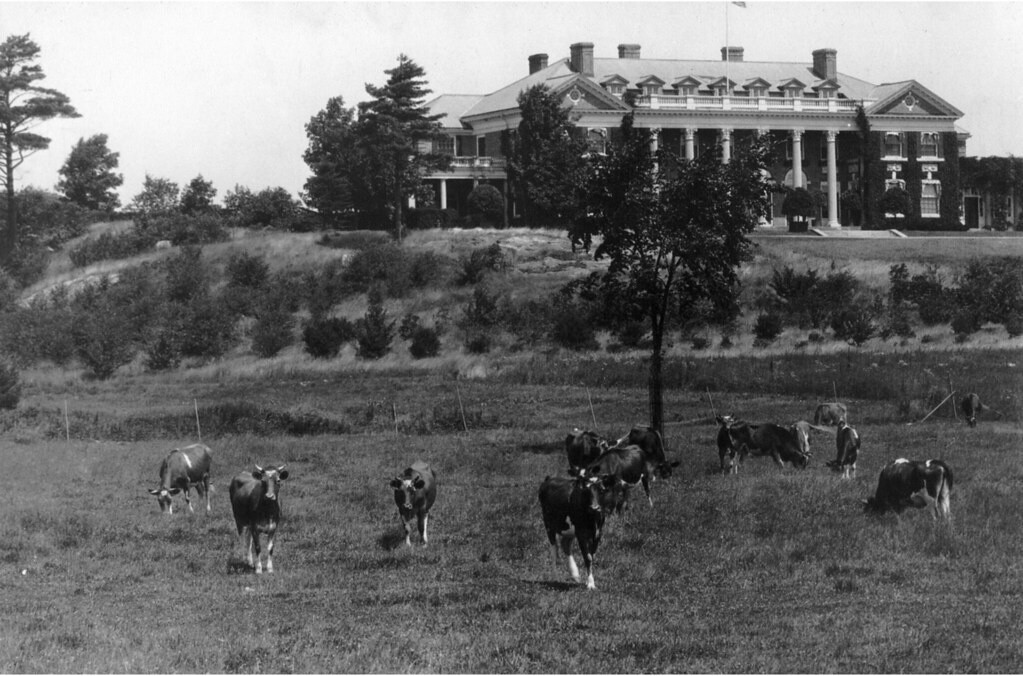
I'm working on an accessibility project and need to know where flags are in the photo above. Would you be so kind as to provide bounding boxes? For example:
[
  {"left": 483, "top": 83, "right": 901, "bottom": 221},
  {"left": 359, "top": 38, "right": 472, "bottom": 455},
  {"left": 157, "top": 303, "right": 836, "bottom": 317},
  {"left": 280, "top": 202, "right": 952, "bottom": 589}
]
[{"left": 732, "top": 1, "right": 747, "bottom": 8}]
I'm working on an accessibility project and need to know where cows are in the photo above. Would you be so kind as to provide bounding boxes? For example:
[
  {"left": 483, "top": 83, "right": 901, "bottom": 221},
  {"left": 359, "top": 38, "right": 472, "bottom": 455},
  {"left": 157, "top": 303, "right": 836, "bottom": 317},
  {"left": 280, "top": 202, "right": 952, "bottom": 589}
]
[
  {"left": 961, "top": 392, "right": 982, "bottom": 429},
  {"left": 147, "top": 442, "right": 214, "bottom": 518},
  {"left": 538, "top": 424, "right": 681, "bottom": 592},
  {"left": 862, "top": 457, "right": 954, "bottom": 520},
  {"left": 715, "top": 412, "right": 814, "bottom": 478},
  {"left": 814, "top": 402, "right": 861, "bottom": 479},
  {"left": 229, "top": 463, "right": 289, "bottom": 575},
  {"left": 390, "top": 460, "right": 437, "bottom": 550}
]
